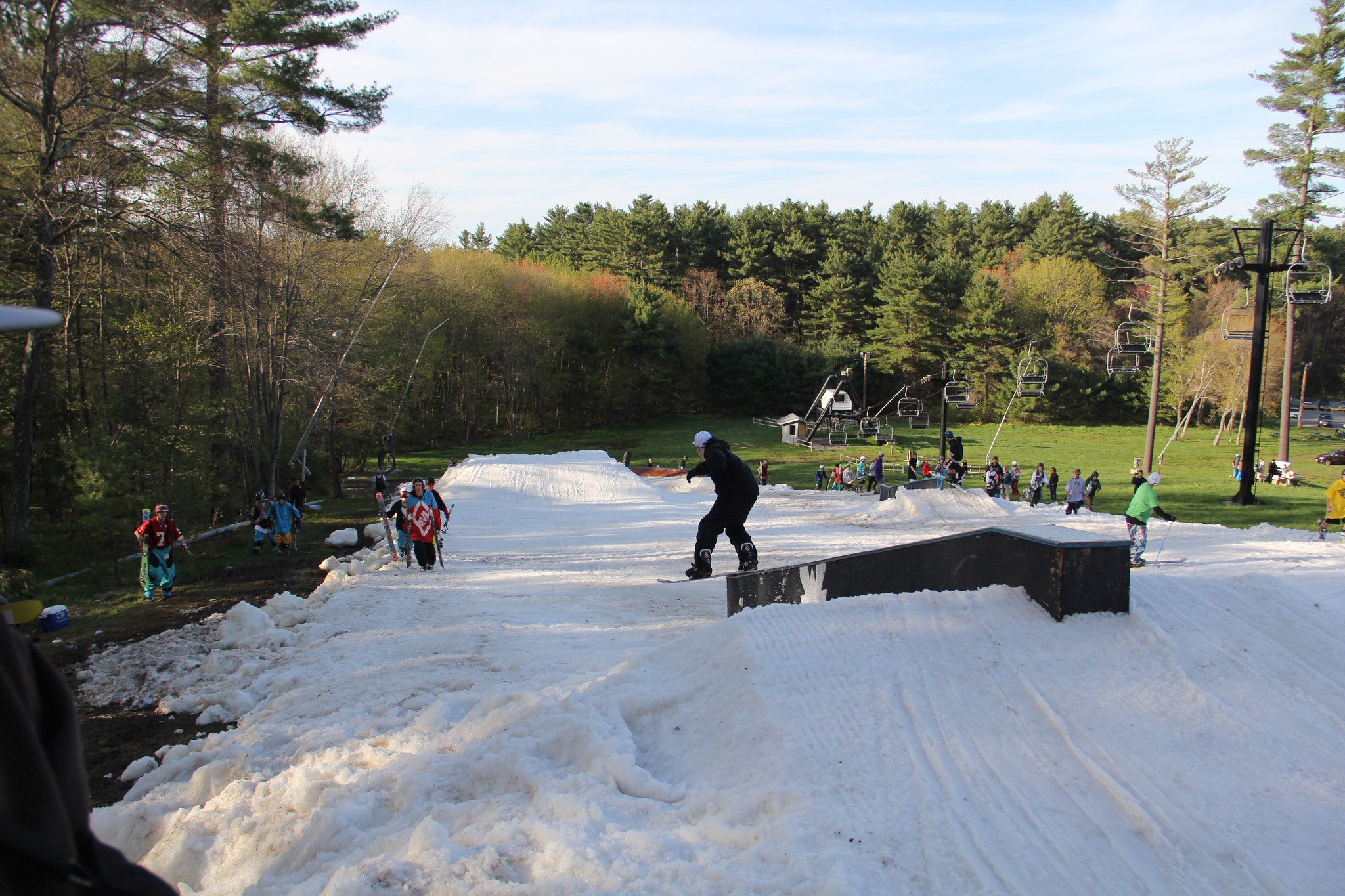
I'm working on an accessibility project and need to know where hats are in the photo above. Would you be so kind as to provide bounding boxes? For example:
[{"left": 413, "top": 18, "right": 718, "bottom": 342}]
[
  {"left": 912, "top": 452, "right": 917, "bottom": 456},
  {"left": 916, "top": 461, "right": 922, "bottom": 467},
  {"left": 684, "top": 455, "right": 688, "bottom": 459},
  {"left": 692, "top": 430, "right": 712, "bottom": 448},
  {"left": 846, "top": 463, "right": 852, "bottom": 466},
  {"left": 1147, "top": 472, "right": 1160, "bottom": 486},
  {"left": 648, "top": 458, "right": 653, "bottom": 461},
  {"left": 1342, "top": 469, "right": 1345, "bottom": 475},
  {"left": 1092, "top": 471, "right": 1099, "bottom": 476},
  {"left": 992, "top": 456, "right": 998, "bottom": 461}
]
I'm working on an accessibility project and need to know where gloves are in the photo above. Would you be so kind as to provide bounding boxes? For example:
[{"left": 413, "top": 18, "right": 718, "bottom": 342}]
[
  {"left": 686, "top": 468, "right": 698, "bottom": 483},
  {"left": 182, "top": 545, "right": 189, "bottom": 552},
  {"left": 1167, "top": 515, "right": 1177, "bottom": 522},
  {"left": 914, "top": 471, "right": 917, "bottom": 475}
]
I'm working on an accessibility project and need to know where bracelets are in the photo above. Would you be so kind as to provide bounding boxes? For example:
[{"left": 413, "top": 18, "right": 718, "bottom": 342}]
[
  {"left": 139, "top": 544, "right": 143, "bottom": 550},
  {"left": 181, "top": 544, "right": 186, "bottom": 548}
]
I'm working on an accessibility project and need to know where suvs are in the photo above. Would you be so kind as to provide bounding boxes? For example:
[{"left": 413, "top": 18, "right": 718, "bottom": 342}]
[
  {"left": 1318, "top": 412, "right": 1333, "bottom": 427},
  {"left": 1299, "top": 396, "right": 1315, "bottom": 409}
]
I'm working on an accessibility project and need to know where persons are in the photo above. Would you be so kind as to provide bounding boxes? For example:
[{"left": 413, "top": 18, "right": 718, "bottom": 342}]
[
  {"left": 406, "top": 477, "right": 442, "bottom": 573},
  {"left": 814, "top": 451, "right": 1101, "bottom": 516},
  {"left": 1231, "top": 453, "right": 1288, "bottom": 483},
  {"left": 1125, "top": 472, "right": 1176, "bottom": 564},
  {"left": 678, "top": 455, "right": 688, "bottom": 469},
  {"left": 424, "top": 477, "right": 450, "bottom": 553},
  {"left": 250, "top": 490, "right": 279, "bottom": 552},
  {"left": 757, "top": 459, "right": 768, "bottom": 485},
  {"left": 1317, "top": 469, "right": 1345, "bottom": 542},
  {"left": 1132, "top": 470, "right": 1147, "bottom": 493},
  {"left": 374, "top": 469, "right": 387, "bottom": 502},
  {"left": 647, "top": 459, "right": 653, "bottom": 467},
  {"left": 380, "top": 487, "right": 414, "bottom": 560},
  {"left": 290, "top": 478, "right": 307, "bottom": 533},
  {"left": 944, "top": 431, "right": 963, "bottom": 481},
  {"left": 134, "top": 504, "right": 188, "bottom": 599},
  {"left": 684, "top": 431, "right": 759, "bottom": 577},
  {"left": 266, "top": 493, "right": 301, "bottom": 554},
  {"left": 448, "top": 459, "right": 457, "bottom": 467}
]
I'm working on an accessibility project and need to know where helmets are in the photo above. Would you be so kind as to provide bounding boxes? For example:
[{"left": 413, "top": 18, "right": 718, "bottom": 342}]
[
  {"left": 154, "top": 504, "right": 169, "bottom": 513},
  {"left": 944, "top": 431, "right": 953, "bottom": 438},
  {"left": 1073, "top": 469, "right": 1081, "bottom": 475},
  {"left": 426, "top": 477, "right": 436, "bottom": 485},
  {"left": 939, "top": 456, "right": 944, "bottom": 460},
  {"left": 277, "top": 493, "right": 286, "bottom": 500},
  {"left": 879, "top": 452, "right": 885, "bottom": 456},
  {"left": 1037, "top": 464, "right": 1044, "bottom": 468},
  {"left": 398, "top": 487, "right": 411, "bottom": 494},
  {"left": 255, "top": 491, "right": 265, "bottom": 500},
  {"left": 1237, "top": 454, "right": 1241, "bottom": 457},
  {"left": 874, "top": 458, "right": 878, "bottom": 461},
  {"left": 1235, "top": 453, "right": 1240, "bottom": 456},
  {"left": 989, "top": 464, "right": 994, "bottom": 468},
  {"left": 763, "top": 459, "right": 767, "bottom": 462},
  {"left": 818, "top": 465, "right": 824, "bottom": 469},
  {"left": 292, "top": 477, "right": 300, "bottom": 480},
  {"left": 859, "top": 456, "right": 866, "bottom": 460},
  {"left": 1011, "top": 461, "right": 1017, "bottom": 466},
  {"left": 413, "top": 477, "right": 424, "bottom": 485}
]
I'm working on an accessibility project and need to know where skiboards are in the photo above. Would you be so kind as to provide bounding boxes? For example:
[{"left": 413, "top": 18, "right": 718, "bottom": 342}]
[
  {"left": 406, "top": 507, "right": 445, "bottom": 569},
  {"left": 759, "top": 461, "right": 762, "bottom": 485},
  {"left": 1130, "top": 558, "right": 1190, "bottom": 567},
  {"left": 1083, "top": 480, "right": 1092, "bottom": 499},
  {"left": 839, "top": 464, "right": 842, "bottom": 492},
  {"left": 816, "top": 467, "right": 831, "bottom": 490},
  {"left": 847, "top": 465, "right": 877, "bottom": 493},
  {"left": 1019, "top": 476, "right": 1042, "bottom": 502},
  {"left": 929, "top": 470, "right": 969, "bottom": 492},
  {"left": 375, "top": 492, "right": 398, "bottom": 561},
  {"left": 139, "top": 507, "right": 150, "bottom": 587}
]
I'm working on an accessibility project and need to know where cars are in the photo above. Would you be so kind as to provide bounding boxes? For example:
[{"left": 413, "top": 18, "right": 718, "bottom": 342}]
[
  {"left": 1337, "top": 400, "right": 1345, "bottom": 408},
  {"left": 1315, "top": 449, "right": 1345, "bottom": 465},
  {"left": 1318, "top": 399, "right": 1331, "bottom": 411},
  {"left": 1289, "top": 407, "right": 1300, "bottom": 419}
]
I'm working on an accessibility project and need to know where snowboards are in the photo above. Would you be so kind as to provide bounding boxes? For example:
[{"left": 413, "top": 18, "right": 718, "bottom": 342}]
[{"left": 657, "top": 567, "right": 776, "bottom": 583}]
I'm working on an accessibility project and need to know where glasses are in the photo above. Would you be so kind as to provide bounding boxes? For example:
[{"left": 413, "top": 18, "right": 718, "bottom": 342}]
[
  {"left": 1051, "top": 470, "right": 1054, "bottom": 471},
  {"left": 913, "top": 454, "right": 917, "bottom": 456},
  {"left": 400, "top": 491, "right": 410, "bottom": 498},
  {"left": 427, "top": 483, "right": 434, "bottom": 487}
]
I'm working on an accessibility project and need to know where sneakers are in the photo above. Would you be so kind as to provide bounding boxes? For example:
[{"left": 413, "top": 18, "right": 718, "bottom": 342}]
[
  {"left": 1129, "top": 561, "right": 1145, "bottom": 568},
  {"left": 250, "top": 545, "right": 261, "bottom": 554},
  {"left": 950, "top": 474, "right": 957, "bottom": 483},
  {"left": 427, "top": 564, "right": 434, "bottom": 570},
  {"left": 858, "top": 488, "right": 864, "bottom": 493},
  {"left": 874, "top": 492, "right": 877, "bottom": 494},
  {"left": 1318, "top": 529, "right": 1327, "bottom": 541},
  {"left": 1033, "top": 503, "right": 1038, "bottom": 508},
  {"left": 855, "top": 488, "right": 858, "bottom": 493},
  {"left": 420, "top": 566, "right": 427, "bottom": 573},
  {"left": 277, "top": 549, "right": 284, "bottom": 556},
  {"left": 272, "top": 544, "right": 279, "bottom": 553},
  {"left": 163, "top": 592, "right": 172, "bottom": 598},
  {"left": 944, "top": 472, "right": 952, "bottom": 480},
  {"left": 685, "top": 549, "right": 712, "bottom": 577},
  {"left": 147, "top": 594, "right": 153, "bottom": 600},
  {"left": 285, "top": 547, "right": 291, "bottom": 555},
  {"left": 1339, "top": 528, "right": 1345, "bottom": 542},
  {"left": 737, "top": 542, "right": 758, "bottom": 571},
  {"left": 876, "top": 492, "right": 880, "bottom": 494},
  {"left": 399, "top": 548, "right": 405, "bottom": 561},
  {"left": 404, "top": 547, "right": 409, "bottom": 561}
]
